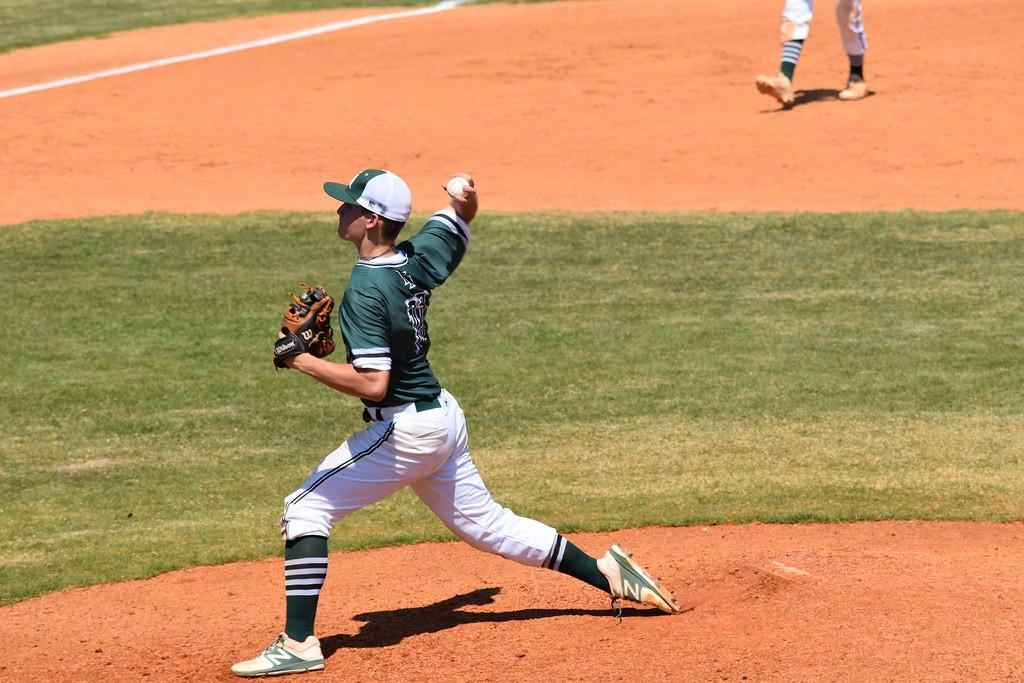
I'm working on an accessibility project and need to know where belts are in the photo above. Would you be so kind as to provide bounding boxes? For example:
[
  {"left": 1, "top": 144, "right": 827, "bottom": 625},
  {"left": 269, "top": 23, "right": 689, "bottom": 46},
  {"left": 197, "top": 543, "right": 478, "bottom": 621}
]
[{"left": 362, "top": 395, "right": 442, "bottom": 423}]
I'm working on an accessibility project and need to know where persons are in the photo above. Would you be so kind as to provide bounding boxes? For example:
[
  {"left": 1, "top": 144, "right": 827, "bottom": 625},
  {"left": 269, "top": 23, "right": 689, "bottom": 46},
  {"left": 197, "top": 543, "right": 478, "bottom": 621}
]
[
  {"left": 229, "top": 169, "right": 679, "bottom": 677},
  {"left": 756, "top": 0, "right": 868, "bottom": 108}
]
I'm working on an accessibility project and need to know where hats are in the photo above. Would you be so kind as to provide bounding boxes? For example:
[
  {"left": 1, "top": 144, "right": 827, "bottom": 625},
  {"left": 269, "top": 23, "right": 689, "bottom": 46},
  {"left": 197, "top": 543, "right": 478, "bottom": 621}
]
[{"left": 323, "top": 169, "right": 411, "bottom": 222}]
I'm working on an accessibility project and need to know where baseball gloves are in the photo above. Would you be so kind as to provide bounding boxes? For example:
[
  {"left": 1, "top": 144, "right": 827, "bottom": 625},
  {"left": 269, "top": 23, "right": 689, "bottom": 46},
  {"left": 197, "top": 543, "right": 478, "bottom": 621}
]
[{"left": 273, "top": 285, "right": 335, "bottom": 368}]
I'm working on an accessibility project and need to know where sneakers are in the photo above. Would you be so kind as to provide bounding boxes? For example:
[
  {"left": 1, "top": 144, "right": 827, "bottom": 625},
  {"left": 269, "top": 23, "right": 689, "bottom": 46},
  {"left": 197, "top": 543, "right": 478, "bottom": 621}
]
[
  {"left": 756, "top": 72, "right": 795, "bottom": 106},
  {"left": 231, "top": 632, "right": 325, "bottom": 676},
  {"left": 597, "top": 545, "right": 679, "bottom": 619},
  {"left": 838, "top": 74, "right": 867, "bottom": 99}
]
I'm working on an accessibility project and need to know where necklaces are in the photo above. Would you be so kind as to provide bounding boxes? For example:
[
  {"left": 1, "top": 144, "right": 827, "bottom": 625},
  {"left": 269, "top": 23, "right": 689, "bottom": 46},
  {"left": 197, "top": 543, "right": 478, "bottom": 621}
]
[{"left": 357, "top": 244, "right": 395, "bottom": 261}]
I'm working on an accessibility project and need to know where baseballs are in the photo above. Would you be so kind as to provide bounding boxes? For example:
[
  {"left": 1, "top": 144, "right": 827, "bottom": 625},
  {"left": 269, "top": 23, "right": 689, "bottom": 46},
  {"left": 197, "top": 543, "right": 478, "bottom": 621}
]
[{"left": 447, "top": 176, "right": 470, "bottom": 200}]
